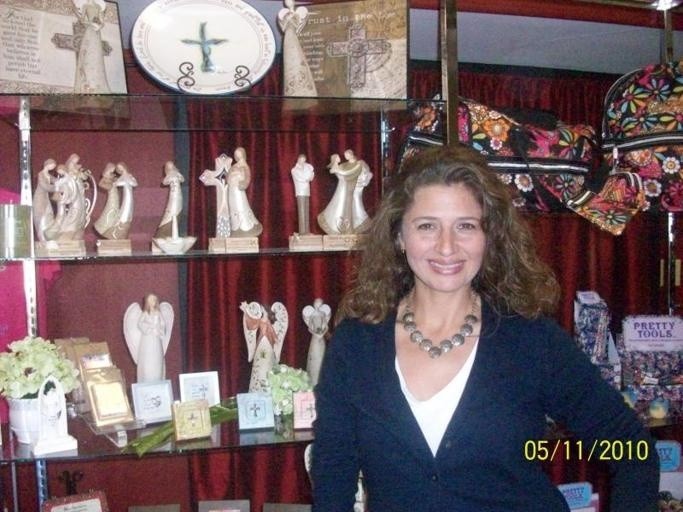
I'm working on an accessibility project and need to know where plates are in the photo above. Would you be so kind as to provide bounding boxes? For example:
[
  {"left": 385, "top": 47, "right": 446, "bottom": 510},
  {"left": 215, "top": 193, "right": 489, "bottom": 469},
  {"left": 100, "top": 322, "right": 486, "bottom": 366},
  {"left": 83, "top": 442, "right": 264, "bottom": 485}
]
[{"left": 131, "top": 0, "right": 278, "bottom": 97}]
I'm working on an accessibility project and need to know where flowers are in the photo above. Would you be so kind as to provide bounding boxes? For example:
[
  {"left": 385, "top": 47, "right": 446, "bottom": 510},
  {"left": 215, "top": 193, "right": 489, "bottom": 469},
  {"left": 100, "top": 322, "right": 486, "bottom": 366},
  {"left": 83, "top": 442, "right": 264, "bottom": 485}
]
[
  {"left": 0, "top": 333, "right": 82, "bottom": 399},
  {"left": 119, "top": 360, "right": 313, "bottom": 459}
]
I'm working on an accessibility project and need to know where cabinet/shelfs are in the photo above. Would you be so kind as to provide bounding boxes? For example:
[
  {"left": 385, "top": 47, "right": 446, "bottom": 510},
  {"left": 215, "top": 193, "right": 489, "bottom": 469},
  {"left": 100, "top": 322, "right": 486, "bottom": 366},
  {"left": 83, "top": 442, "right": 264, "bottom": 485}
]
[{"left": 0, "top": 92, "right": 449, "bottom": 512}]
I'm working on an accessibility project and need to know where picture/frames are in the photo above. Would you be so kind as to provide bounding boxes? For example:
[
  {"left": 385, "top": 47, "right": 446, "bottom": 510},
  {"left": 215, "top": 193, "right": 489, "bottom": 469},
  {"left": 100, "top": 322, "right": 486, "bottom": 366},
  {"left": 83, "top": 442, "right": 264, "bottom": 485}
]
[{"left": 54, "top": 336, "right": 316, "bottom": 444}]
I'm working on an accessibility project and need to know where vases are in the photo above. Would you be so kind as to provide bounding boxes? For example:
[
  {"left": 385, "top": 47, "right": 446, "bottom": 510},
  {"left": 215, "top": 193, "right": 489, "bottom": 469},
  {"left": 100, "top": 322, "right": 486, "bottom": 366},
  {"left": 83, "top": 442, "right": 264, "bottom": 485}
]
[{"left": 4, "top": 396, "right": 42, "bottom": 446}]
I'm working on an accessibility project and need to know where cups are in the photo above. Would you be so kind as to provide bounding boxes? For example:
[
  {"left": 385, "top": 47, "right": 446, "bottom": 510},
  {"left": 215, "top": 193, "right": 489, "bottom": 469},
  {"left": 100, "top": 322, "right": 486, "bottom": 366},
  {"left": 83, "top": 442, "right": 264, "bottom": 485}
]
[{"left": 0, "top": 204, "right": 31, "bottom": 259}]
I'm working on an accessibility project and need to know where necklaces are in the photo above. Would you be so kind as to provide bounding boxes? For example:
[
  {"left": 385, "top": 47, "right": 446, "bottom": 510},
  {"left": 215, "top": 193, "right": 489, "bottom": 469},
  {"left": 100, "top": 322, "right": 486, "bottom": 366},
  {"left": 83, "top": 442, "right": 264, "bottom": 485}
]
[{"left": 400, "top": 286, "right": 480, "bottom": 360}]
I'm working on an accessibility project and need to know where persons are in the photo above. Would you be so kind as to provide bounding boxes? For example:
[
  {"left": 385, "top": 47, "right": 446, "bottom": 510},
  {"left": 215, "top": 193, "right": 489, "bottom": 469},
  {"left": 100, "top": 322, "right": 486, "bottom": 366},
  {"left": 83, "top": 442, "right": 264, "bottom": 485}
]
[
  {"left": 274, "top": 0, "right": 320, "bottom": 111},
  {"left": 290, "top": 154, "right": 315, "bottom": 235},
  {"left": 155, "top": 160, "right": 184, "bottom": 238},
  {"left": 308, "top": 142, "right": 660, "bottom": 510},
  {"left": 228, "top": 147, "right": 263, "bottom": 237},
  {"left": 317, "top": 149, "right": 371, "bottom": 235},
  {"left": 33, "top": 154, "right": 88, "bottom": 242},
  {"left": 237, "top": 302, "right": 283, "bottom": 390},
  {"left": 71, "top": 1, "right": 117, "bottom": 111},
  {"left": 134, "top": 291, "right": 168, "bottom": 381},
  {"left": 96, "top": 162, "right": 139, "bottom": 241},
  {"left": 305, "top": 297, "right": 330, "bottom": 386}
]
